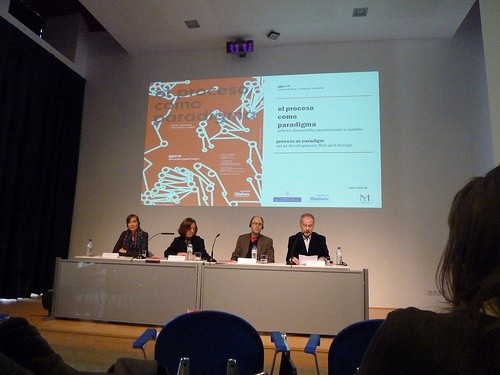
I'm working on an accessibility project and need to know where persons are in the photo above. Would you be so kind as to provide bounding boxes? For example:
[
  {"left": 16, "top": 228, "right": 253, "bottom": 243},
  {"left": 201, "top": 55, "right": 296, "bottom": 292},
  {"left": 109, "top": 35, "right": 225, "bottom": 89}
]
[
  {"left": 285, "top": 214, "right": 330, "bottom": 265},
  {"left": 164, "top": 218, "right": 217, "bottom": 262},
  {"left": 355, "top": 165, "right": 500, "bottom": 375},
  {"left": 230, "top": 216, "right": 274, "bottom": 263},
  {"left": 112, "top": 214, "right": 149, "bottom": 259}
]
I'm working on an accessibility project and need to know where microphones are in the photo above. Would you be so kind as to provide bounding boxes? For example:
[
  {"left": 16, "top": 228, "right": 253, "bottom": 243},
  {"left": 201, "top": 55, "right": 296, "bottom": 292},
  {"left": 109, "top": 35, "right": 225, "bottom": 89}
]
[
  {"left": 137, "top": 233, "right": 174, "bottom": 259},
  {"left": 286, "top": 235, "right": 301, "bottom": 265},
  {"left": 207, "top": 234, "right": 220, "bottom": 262}
]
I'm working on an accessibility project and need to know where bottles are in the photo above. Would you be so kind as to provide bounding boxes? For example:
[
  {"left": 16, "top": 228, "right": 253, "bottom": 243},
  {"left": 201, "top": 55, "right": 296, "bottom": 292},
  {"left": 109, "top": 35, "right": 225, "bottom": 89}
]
[
  {"left": 336, "top": 247, "right": 342, "bottom": 265},
  {"left": 87, "top": 239, "right": 93, "bottom": 257},
  {"left": 186, "top": 243, "right": 193, "bottom": 260},
  {"left": 251, "top": 245, "right": 258, "bottom": 261}
]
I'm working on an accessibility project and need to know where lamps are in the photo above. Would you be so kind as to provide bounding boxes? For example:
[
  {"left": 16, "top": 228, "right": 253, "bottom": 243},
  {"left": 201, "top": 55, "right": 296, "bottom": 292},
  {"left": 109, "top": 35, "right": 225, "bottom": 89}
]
[
  {"left": 225, "top": 39, "right": 254, "bottom": 58},
  {"left": 268, "top": 31, "right": 280, "bottom": 40}
]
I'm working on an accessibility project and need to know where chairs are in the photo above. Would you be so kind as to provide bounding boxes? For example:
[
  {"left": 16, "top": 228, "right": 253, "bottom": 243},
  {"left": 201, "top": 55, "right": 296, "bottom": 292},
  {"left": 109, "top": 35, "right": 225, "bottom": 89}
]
[
  {"left": 304, "top": 319, "right": 384, "bottom": 375},
  {"left": 133, "top": 309, "right": 290, "bottom": 375}
]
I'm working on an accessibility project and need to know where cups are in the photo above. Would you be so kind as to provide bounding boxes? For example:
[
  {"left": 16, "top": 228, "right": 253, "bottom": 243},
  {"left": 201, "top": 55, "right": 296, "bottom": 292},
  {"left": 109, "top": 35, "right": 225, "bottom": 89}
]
[
  {"left": 139, "top": 250, "right": 146, "bottom": 257},
  {"left": 260, "top": 255, "right": 268, "bottom": 264},
  {"left": 195, "top": 252, "right": 201, "bottom": 261}
]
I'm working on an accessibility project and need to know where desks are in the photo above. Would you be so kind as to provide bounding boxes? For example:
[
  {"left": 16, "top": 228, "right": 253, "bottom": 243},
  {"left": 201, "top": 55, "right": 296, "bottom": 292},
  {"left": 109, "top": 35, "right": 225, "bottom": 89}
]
[{"left": 51, "top": 257, "right": 369, "bottom": 336}]
